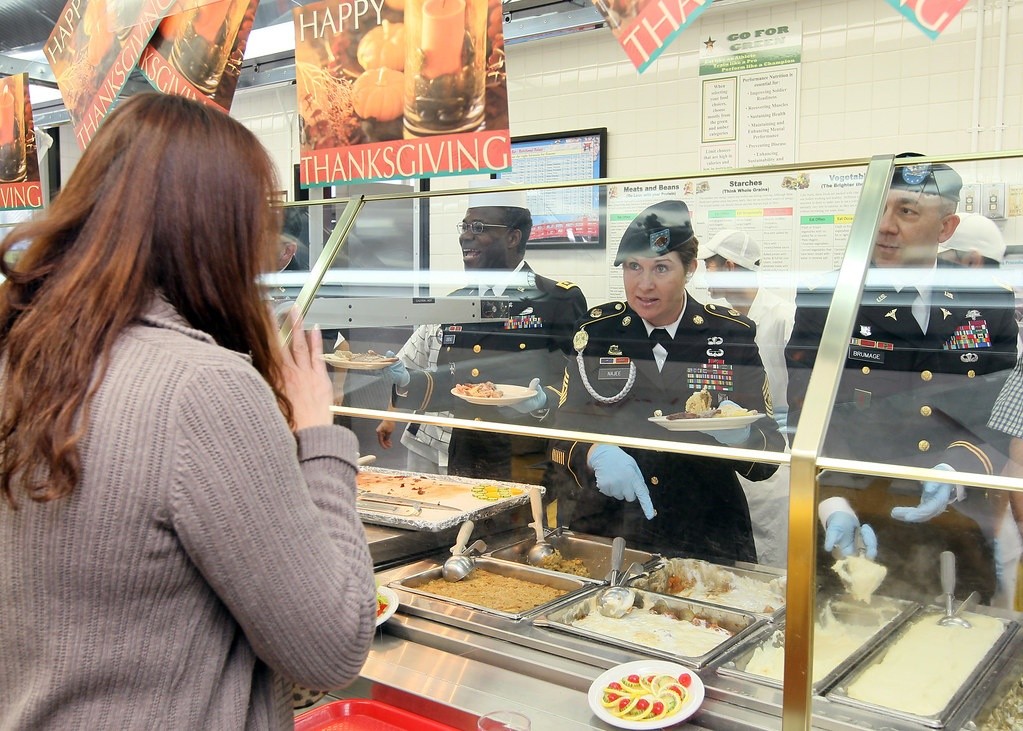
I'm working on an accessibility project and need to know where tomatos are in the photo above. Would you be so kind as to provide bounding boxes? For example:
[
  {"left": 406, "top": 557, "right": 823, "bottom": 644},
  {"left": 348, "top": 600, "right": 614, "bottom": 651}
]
[{"left": 609, "top": 673, "right": 691, "bottom": 713}]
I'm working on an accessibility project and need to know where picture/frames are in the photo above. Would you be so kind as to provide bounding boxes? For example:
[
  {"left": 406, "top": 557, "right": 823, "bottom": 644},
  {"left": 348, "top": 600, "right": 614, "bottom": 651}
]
[{"left": 489, "top": 126, "right": 608, "bottom": 251}]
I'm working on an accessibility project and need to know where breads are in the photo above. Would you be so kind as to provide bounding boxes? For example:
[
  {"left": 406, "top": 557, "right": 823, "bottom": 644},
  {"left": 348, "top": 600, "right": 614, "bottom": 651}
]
[{"left": 350, "top": 19, "right": 404, "bottom": 121}]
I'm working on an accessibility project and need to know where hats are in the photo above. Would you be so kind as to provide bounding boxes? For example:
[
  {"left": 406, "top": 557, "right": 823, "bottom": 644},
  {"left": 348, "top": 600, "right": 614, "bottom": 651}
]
[
  {"left": 940, "top": 212, "right": 1006, "bottom": 263},
  {"left": 892, "top": 152, "right": 963, "bottom": 201},
  {"left": 467, "top": 176, "right": 530, "bottom": 209},
  {"left": 696, "top": 231, "right": 763, "bottom": 271},
  {"left": 267, "top": 198, "right": 303, "bottom": 239},
  {"left": 614, "top": 200, "right": 694, "bottom": 269}
]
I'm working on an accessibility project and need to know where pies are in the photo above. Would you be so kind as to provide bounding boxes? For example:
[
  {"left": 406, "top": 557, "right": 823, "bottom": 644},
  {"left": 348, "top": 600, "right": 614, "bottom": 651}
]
[
  {"left": 335, "top": 350, "right": 399, "bottom": 362},
  {"left": 456, "top": 381, "right": 504, "bottom": 397}
]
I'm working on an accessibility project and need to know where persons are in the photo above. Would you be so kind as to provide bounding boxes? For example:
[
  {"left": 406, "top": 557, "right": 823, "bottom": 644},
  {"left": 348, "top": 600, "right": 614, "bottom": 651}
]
[
  {"left": 376, "top": 299, "right": 457, "bottom": 476},
  {"left": 697, "top": 229, "right": 794, "bottom": 568},
  {"left": 784, "top": 153, "right": 1018, "bottom": 607},
  {"left": 937, "top": 212, "right": 1008, "bottom": 275},
  {"left": 0, "top": 92, "right": 375, "bottom": 731},
  {"left": 369, "top": 179, "right": 588, "bottom": 531},
  {"left": 985, "top": 350, "right": 1023, "bottom": 541},
  {"left": 269, "top": 200, "right": 351, "bottom": 442},
  {"left": 548, "top": 200, "right": 785, "bottom": 567}
]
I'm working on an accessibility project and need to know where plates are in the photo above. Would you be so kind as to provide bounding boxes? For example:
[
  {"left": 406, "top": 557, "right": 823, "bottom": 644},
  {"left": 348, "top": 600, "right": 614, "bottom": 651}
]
[
  {"left": 450, "top": 384, "right": 538, "bottom": 406},
  {"left": 646, "top": 413, "right": 768, "bottom": 432},
  {"left": 323, "top": 353, "right": 395, "bottom": 370},
  {"left": 374, "top": 586, "right": 401, "bottom": 627},
  {"left": 587, "top": 659, "right": 706, "bottom": 730}
]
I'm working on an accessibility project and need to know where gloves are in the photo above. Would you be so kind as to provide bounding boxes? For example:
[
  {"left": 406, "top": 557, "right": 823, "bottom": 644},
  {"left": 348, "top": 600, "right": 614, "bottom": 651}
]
[
  {"left": 590, "top": 447, "right": 654, "bottom": 520},
  {"left": 891, "top": 468, "right": 955, "bottom": 521},
  {"left": 701, "top": 399, "right": 750, "bottom": 447},
  {"left": 824, "top": 513, "right": 879, "bottom": 561},
  {"left": 384, "top": 351, "right": 412, "bottom": 390},
  {"left": 508, "top": 378, "right": 546, "bottom": 414}
]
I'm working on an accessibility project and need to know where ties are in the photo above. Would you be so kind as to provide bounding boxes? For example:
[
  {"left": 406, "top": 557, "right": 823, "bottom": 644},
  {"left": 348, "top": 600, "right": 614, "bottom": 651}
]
[
  {"left": 897, "top": 286, "right": 920, "bottom": 312},
  {"left": 484, "top": 290, "right": 494, "bottom": 295},
  {"left": 649, "top": 328, "right": 673, "bottom": 352}
]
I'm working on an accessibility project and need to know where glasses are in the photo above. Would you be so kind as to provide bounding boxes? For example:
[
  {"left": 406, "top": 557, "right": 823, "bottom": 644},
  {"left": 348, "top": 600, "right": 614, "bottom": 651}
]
[{"left": 457, "top": 222, "right": 508, "bottom": 234}]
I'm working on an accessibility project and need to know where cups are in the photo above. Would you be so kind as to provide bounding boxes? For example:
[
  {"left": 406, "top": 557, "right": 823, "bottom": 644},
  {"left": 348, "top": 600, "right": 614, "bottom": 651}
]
[
  {"left": 477, "top": 709, "right": 533, "bottom": 731},
  {"left": 404, "top": 0, "right": 490, "bottom": 139},
  {"left": 166, "top": 0, "right": 250, "bottom": 100},
  {"left": 0, "top": 73, "right": 28, "bottom": 185}
]
